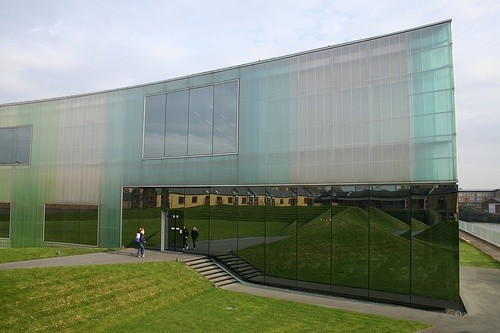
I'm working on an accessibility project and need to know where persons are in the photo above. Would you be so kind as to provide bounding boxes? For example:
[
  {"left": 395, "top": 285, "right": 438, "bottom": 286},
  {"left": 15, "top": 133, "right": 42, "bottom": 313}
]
[
  {"left": 190, "top": 226, "right": 199, "bottom": 251},
  {"left": 136, "top": 227, "right": 147, "bottom": 258},
  {"left": 183, "top": 226, "right": 189, "bottom": 250}
]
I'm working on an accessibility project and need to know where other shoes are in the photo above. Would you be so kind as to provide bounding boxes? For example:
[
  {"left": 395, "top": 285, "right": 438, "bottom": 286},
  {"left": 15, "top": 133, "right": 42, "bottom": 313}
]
[
  {"left": 137, "top": 256, "right": 140, "bottom": 257},
  {"left": 183, "top": 246, "right": 188, "bottom": 250},
  {"left": 193, "top": 248, "right": 195, "bottom": 250},
  {"left": 142, "top": 254, "right": 145, "bottom": 257}
]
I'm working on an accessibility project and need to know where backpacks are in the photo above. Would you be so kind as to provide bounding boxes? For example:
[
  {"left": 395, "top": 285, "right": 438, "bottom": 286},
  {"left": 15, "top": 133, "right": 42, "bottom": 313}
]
[{"left": 136, "top": 233, "right": 140, "bottom": 243}]
